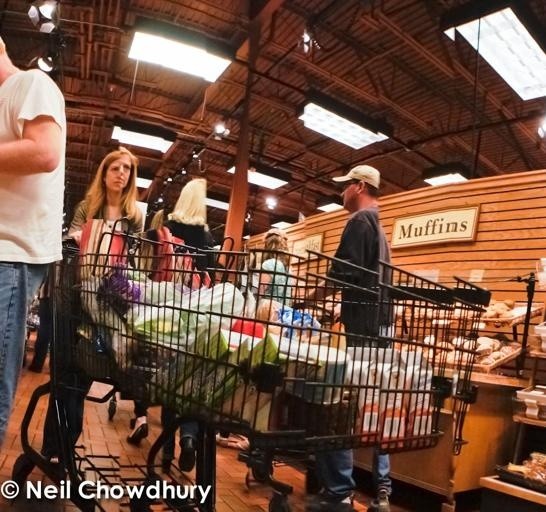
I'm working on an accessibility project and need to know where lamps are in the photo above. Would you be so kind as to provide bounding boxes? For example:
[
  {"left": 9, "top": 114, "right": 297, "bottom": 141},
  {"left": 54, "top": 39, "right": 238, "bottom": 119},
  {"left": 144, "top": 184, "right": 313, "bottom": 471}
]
[
  {"left": 315, "top": 194, "right": 344, "bottom": 212},
  {"left": 422, "top": 162, "right": 481, "bottom": 186},
  {"left": 110, "top": 116, "right": 177, "bottom": 154},
  {"left": 127, "top": 16, "right": 235, "bottom": 83},
  {"left": 226, "top": 158, "right": 291, "bottom": 190},
  {"left": 439, "top": 0, "right": 546, "bottom": 102},
  {"left": 295, "top": 92, "right": 392, "bottom": 151},
  {"left": 304, "top": 31, "right": 314, "bottom": 43},
  {"left": 192, "top": 144, "right": 206, "bottom": 158},
  {"left": 38, "top": 55, "right": 53, "bottom": 73},
  {"left": 135, "top": 170, "right": 154, "bottom": 189},
  {"left": 206, "top": 190, "right": 229, "bottom": 211},
  {"left": 269, "top": 215, "right": 298, "bottom": 228}
]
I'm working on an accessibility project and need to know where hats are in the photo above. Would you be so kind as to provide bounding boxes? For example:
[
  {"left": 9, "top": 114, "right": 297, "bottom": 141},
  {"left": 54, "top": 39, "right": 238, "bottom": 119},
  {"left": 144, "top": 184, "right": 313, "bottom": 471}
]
[{"left": 331, "top": 164, "right": 381, "bottom": 189}]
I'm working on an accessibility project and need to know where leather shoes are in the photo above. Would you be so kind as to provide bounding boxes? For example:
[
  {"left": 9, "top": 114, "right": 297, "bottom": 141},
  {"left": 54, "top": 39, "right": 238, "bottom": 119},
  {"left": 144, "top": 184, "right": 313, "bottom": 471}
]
[
  {"left": 178, "top": 438, "right": 195, "bottom": 472},
  {"left": 126, "top": 422, "right": 149, "bottom": 445}
]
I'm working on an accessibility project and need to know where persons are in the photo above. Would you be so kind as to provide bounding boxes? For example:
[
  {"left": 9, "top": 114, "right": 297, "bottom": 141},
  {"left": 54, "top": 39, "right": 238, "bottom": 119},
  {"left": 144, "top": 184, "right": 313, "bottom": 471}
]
[
  {"left": 26, "top": 148, "right": 294, "bottom": 479},
  {"left": 286, "top": 165, "right": 395, "bottom": 511},
  {"left": 0, "top": 34, "right": 70, "bottom": 448}
]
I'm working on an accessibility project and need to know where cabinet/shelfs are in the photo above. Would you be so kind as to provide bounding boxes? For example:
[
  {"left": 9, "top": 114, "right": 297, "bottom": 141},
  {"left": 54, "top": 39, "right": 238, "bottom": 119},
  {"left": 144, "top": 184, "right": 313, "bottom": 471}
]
[{"left": 479, "top": 349, "right": 546, "bottom": 506}]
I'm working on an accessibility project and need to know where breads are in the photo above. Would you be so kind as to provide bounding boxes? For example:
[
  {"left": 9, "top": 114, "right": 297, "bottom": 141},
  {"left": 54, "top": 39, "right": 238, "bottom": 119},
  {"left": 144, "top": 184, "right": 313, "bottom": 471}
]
[
  {"left": 255, "top": 302, "right": 278, "bottom": 325},
  {"left": 395, "top": 299, "right": 515, "bottom": 364}
]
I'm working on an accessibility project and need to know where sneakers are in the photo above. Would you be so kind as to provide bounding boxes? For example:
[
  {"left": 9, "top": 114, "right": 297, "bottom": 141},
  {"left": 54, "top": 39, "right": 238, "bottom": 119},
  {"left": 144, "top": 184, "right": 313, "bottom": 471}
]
[
  {"left": 305, "top": 495, "right": 352, "bottom": 512},
  {"left": 368, "top": 489, "right": 390, "bottom": 512}
]
